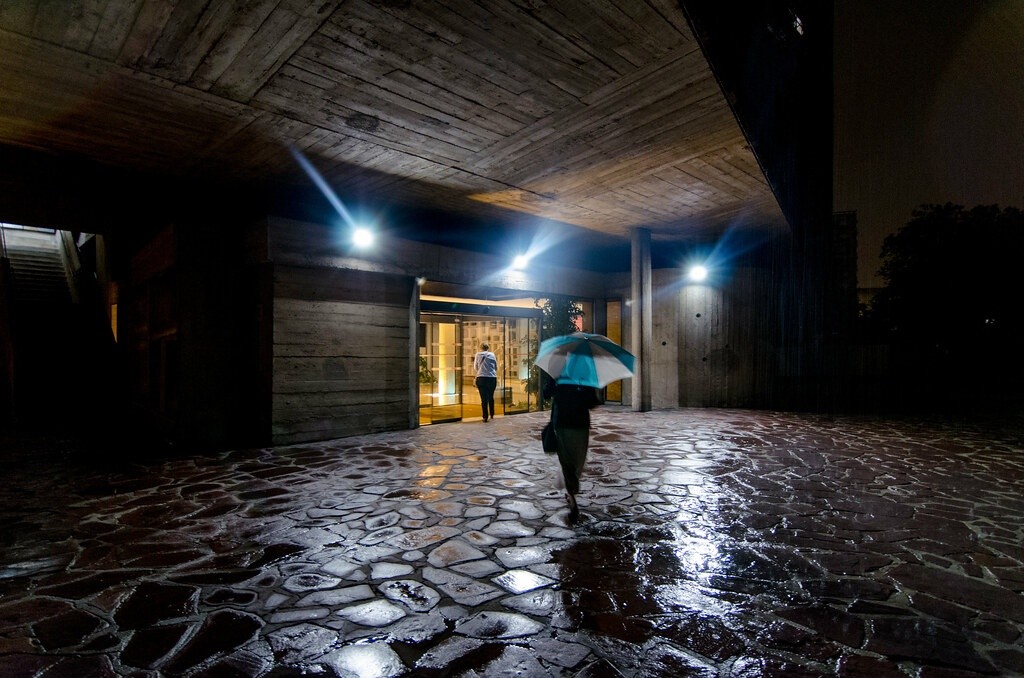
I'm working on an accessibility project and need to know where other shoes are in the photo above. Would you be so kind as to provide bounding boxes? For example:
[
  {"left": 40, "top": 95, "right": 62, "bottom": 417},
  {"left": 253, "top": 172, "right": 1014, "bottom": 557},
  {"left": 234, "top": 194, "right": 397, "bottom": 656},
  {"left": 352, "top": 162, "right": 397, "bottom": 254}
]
[
  {"left": 563, "top": 492, "right": 579, "bottom": 515},
  {"left": 482, "top": 417, "right": 488, "bottom": 423}
]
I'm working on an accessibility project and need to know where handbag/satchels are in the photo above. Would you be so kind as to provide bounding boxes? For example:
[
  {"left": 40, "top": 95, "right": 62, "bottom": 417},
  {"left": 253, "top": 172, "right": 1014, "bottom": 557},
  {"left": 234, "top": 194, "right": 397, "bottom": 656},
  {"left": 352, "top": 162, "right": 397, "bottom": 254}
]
[{"left": 540, "top": 420, "right": 557, "bottom": 455}]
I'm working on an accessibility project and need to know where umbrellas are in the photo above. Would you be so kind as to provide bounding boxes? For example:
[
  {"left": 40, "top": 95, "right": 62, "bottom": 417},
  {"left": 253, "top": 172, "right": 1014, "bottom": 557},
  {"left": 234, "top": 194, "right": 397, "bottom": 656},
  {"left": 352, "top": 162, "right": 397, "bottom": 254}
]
[{"left": 534, "top": 331, "right": 637, "bottom": 389}]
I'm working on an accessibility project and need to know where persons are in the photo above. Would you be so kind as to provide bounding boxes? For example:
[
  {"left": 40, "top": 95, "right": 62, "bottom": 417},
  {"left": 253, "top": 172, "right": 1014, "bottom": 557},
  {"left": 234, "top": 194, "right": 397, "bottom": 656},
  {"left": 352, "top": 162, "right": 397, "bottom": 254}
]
[
  {"left": 473, "top": 341, "right": 497, "bottom": 422},
  {"left": 541, "top": 370, "right": 606, "bottom": 525}
]
